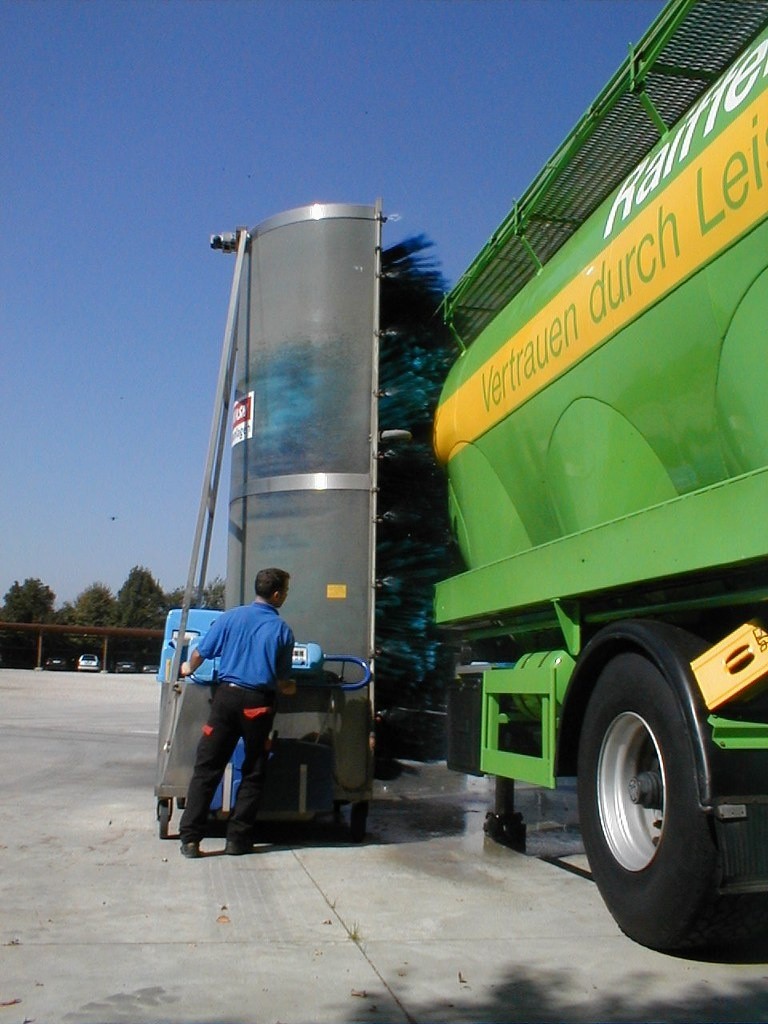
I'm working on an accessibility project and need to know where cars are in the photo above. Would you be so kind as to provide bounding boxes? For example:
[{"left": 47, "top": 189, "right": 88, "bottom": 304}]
[
  {"left": 116, "top": 658, "right": 137, "bottom": 675},
  {"left": 141, "top": 661, "right": 159, "bottom": 673},
  {"left": 46, "top": 655, "right": 74, "bottom": 671}
]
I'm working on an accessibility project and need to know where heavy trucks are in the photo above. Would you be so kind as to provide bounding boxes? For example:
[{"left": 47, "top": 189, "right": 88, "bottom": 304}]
[{"left": 425, "top": 0, "right": 768, "bottom": 960}]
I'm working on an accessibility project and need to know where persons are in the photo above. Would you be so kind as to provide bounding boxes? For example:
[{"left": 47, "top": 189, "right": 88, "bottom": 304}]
[{"left": 180, "top": 568, "right": 295, "bottom": 857}]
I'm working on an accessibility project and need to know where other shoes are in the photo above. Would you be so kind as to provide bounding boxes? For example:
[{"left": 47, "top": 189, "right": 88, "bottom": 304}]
[
  {"left": 181, "top": 841, "right": 199, "bottom": 858},
  {"left": 224, "top": 839, "right": 253, "bottom": 855}
]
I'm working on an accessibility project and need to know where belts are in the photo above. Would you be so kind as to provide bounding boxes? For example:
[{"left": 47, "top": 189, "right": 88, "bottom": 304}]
[{"left": 229, "top": 682, "right": 257, "bottom": 693}]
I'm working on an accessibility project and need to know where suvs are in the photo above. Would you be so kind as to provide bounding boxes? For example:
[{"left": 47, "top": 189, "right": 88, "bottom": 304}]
[{"left": 77, "top": 654, "right": 102, "bottom": 672}]
